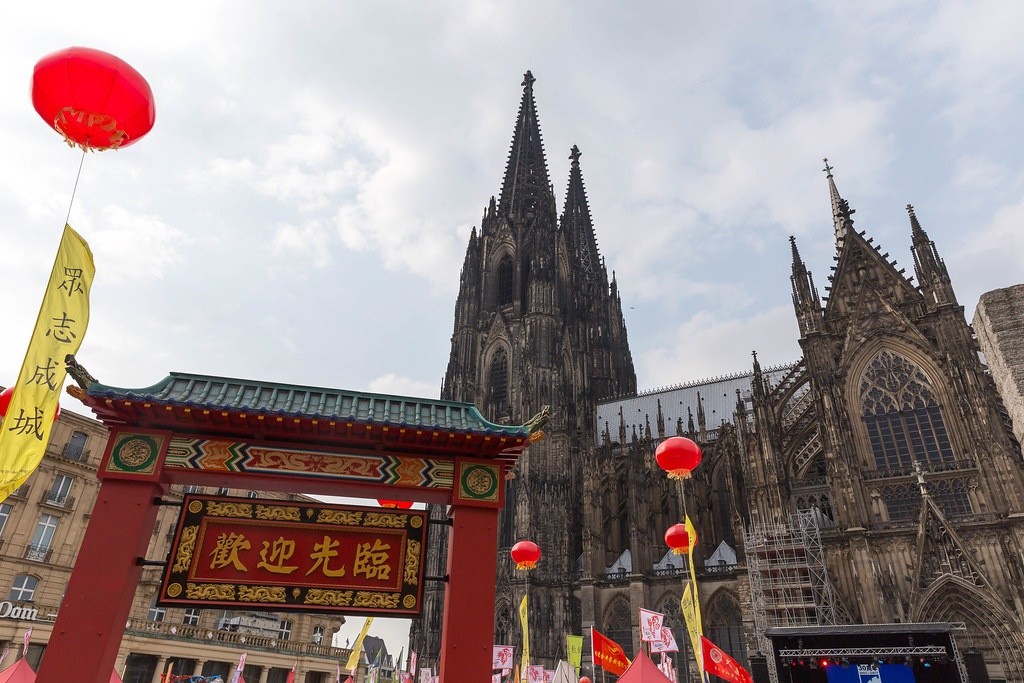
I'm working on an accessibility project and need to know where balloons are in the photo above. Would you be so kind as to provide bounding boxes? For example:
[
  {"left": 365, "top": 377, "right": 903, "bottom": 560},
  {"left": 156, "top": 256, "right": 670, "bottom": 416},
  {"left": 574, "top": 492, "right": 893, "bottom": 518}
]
[
  {"left": 511, "top": 539, "right": 540, "bottom": 570},
  {"left": 655, "top": 436, "right": 701, "bottom": 478},
  {"left": 375, "top": 498, "right": 414, "bottom": 509},
  {"left": 666, "top": 524, "right": 699, "bottom": 556},
  {"left": 0, "top": 386, "right": 61, "bottom": 425},
  {"left": 33, "top": 46, "right": 156, "bottom": 150}
]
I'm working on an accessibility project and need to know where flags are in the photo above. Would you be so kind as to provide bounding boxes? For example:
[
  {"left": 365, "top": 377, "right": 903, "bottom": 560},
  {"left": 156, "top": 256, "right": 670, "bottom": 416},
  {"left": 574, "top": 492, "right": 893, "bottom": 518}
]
[
  {"left": 1, "top": 224, "right": 97, "bottom": 510},
  {"left": 233, "top": 519, "right": 762, "bottom": 683},
  {"left": 23, "top": 626, "right": 32, "bottom": 660}
]
